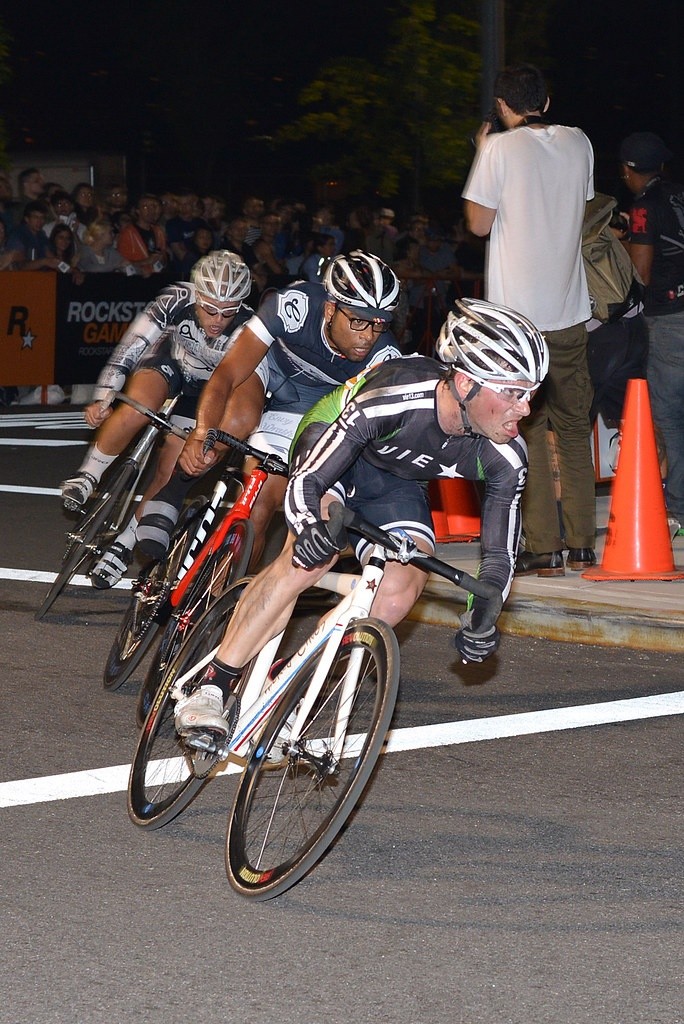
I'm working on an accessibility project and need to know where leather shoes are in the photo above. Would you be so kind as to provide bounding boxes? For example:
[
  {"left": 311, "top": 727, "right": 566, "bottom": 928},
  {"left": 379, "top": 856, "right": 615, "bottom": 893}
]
[
  {"left": 565, "top": 548, "right": 596, "bottom": 570},
  {"left": 513, "top": 549, "right": 565, "bottom": 578}
]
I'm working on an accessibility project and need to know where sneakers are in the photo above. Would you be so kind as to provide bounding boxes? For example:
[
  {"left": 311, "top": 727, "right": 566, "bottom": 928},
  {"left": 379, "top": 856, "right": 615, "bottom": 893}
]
[
  {"left": 89, "top": 541, "right": 133, "bottom": 589},
  {"left": 58, "top": 471, "right": 97, "bottom": 505},
  {"left": 174, "top": 685, "right": 228, "bottom": 737},
  {"left": 133, "top": 498, "right": 180, "bottom": 561},
  {"left": 259, "top": 658, "right": 305, "bottom": 764}
]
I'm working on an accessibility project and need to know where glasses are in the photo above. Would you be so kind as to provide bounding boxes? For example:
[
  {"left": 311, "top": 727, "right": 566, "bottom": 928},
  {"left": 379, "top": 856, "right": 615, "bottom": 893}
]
[
  {"left": 54, "top": 201, "right": 70, "bottom": 207},
  {"left": 335, "top": 304, "right": 393, "bottom": 334},
  {"left": 451, "top": 364, "right": 540, "bottom": 403},
  {"left": 108, "top": 192, "right": 121, "bottom": 197},
  {"left": 618, "top": 228, "right": 627, "bottom": 235},
  {"left": 195, "top": 290, "right": 243, "bottom": 318},
  {"left": 79, "top": 192, "right": 91, "bottom": 198}
]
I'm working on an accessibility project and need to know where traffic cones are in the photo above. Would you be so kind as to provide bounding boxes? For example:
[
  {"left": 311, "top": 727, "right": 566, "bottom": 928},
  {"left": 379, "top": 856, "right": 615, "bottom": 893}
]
[{"left": 581, "top": 378, "right": 683, "bottom": 582}]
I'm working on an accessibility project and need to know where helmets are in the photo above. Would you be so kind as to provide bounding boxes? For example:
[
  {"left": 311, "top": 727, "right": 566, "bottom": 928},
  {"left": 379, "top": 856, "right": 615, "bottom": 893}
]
[
  {"left": 608, "top": 214, "right": 628, "bottom": 230},
  {"left": 193, "top": 249, "right": 253, "bottom": 301},
  {"left": 321, "top": 249, "right": 402, "bottom": 309},
  {"left": 433, "top": 296, "right": 550, "bottom": 384}
]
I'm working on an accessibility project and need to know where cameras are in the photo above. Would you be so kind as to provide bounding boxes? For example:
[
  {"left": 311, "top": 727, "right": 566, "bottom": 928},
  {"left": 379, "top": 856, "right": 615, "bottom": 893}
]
[{"left": 472, "top": 112, "right": 505, "bottom": 146}]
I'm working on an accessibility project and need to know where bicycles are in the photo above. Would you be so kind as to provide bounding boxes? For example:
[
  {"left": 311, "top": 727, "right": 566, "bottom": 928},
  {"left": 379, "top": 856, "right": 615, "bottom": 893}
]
[
  {"left": 126, "top": 479, "right": 504, "bottom": 904},
  {"left": 34, "top": 389, "right": 291, "bottom": 728}
]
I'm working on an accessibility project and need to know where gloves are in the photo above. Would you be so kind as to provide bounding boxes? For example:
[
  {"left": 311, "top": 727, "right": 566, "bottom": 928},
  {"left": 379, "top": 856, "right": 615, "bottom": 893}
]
[
  {"left": 292, "top": 519, "right": 349, "bottom": 572},
  {"left": 455, "top": 608, "right": 502, "bottom": 665}
]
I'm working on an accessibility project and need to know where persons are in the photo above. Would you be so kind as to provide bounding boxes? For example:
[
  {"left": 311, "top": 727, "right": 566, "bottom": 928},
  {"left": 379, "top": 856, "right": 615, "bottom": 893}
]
[
  {"left": 176, "top": 296, "right": 552, "bottom": 738},
  {"left": 1, "top": 132, "right": 683, "bottom": 588},
  {"left": 134, "top": 249, "right": 405, "bottom": 676},
  {"left": 463, "top": 61, "right": 598, "bottom": 576}
]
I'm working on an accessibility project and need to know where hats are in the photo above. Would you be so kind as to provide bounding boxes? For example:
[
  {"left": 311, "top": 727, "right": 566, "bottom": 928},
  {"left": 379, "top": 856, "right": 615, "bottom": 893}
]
[
  {"left": 379, "top": 207, "right": 394, "bottom": 217},
  {"left": 610, "top": 131, "right": 669, "bottom": 174}
]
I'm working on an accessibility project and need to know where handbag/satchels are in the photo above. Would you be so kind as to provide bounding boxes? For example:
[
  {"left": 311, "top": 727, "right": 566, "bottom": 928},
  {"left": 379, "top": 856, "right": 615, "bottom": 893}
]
[{"left": 582, "top": 189, "right": 647, "bottom": 321}]
